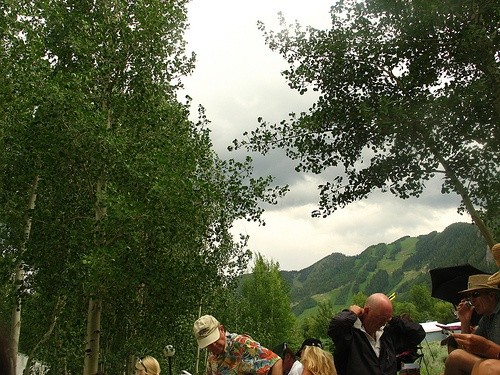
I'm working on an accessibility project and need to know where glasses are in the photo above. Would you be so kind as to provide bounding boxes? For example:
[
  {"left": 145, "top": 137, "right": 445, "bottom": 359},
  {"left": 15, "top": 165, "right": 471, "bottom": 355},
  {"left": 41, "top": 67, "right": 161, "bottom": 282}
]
[
  {"left": 137, "top": 357, "right": 147, "bottom": 372},
  {"left": 468, "top": 290, "right": 492, "bottom": 298}
]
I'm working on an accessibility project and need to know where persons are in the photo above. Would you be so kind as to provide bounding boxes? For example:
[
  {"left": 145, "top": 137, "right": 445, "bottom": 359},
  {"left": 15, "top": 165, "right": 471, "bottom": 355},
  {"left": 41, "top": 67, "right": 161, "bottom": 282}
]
[
  {"left": 272, "top": 342, "right": 304, "bottom": 375},
  {"left": 457, "top": 273, "right": 500, "bottom": 347},
  {"left": 301, "top": 346, "right": 338, "bottom": 375},
  {"left": 443, "top": 243, "right": 500, "bottom": 375},
  {"left": 135, "top": 355, "right": 161, "bottom": 375},
  {"left": 295, "top": 338, "right": 324, "bottom": 361},
  {"left": 327, "top": 292, "right": 426, "bottom": 375},
  {"left": 193, "top": 315, "right": 283, "bottom": 375},
  {"left": 394, "top": 313, "right": 418, "bottom": 372}
]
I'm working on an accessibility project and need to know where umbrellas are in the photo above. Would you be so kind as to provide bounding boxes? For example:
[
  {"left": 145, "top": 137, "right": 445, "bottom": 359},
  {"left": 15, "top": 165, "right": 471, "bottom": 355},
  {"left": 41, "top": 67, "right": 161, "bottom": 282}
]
[
  {"left": 429, "top": 264, "right": 484, "bottom": 304},
  {"left": 419, "top": 321, "right": 444, "bottom": 333},
  {"left": 436, "top": 322, "right": 463, "bottom": 331}
]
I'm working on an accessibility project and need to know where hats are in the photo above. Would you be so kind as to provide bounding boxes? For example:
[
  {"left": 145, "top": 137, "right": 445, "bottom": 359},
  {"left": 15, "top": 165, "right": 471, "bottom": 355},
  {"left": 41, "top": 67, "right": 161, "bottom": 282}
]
[
  {"left": 272, "top": 341, "right": 287, "bottom": 362},
  {"left": 193, "top": 315, "right": 220, "bottom": 350},
  {"left": 296, "top": 338, "right": 322, "bottom": 358},
  {"left": 457, "top": 275, "right": 500, "bottom": 293}
]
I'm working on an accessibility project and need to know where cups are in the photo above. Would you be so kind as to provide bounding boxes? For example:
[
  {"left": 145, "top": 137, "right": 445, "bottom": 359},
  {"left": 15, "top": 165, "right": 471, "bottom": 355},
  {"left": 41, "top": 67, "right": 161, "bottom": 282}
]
[{"left": 457, "top": 301, "right": 472, "bottom": 317}]
